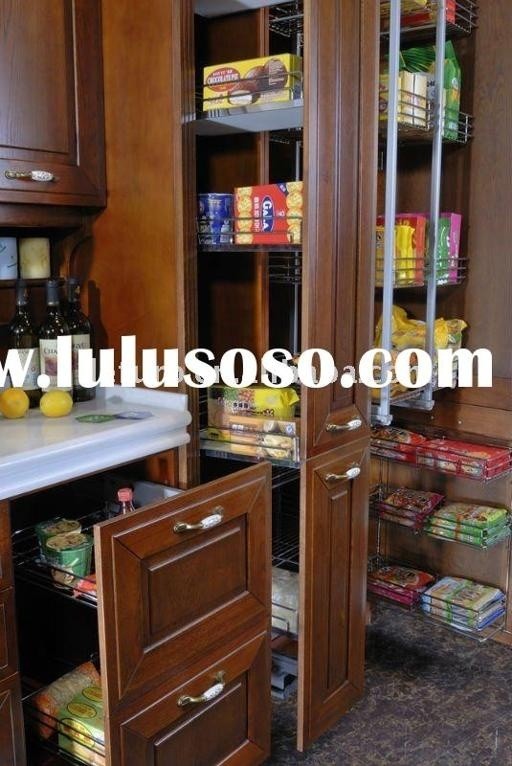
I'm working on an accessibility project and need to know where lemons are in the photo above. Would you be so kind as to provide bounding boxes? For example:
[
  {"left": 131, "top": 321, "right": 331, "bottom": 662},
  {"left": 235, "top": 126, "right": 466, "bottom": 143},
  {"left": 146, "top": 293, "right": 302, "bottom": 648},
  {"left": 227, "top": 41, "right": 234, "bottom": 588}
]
[
  {"left": 0, "top": 387, "right": 30, "bottom": 418},
  {"left": 40, "top": 390, "right": 74, "bottom": 417}
]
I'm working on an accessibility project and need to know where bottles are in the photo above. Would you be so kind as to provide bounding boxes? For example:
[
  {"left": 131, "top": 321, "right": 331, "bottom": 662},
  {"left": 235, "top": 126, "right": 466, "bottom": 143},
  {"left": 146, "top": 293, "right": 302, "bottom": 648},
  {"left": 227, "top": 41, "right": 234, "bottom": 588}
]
[
  {"left": 3, "top": 276, "right": 96, "bottom": 408},
  {"left": 117, "top": 487, "right": 136, "bottom": 517}
]
